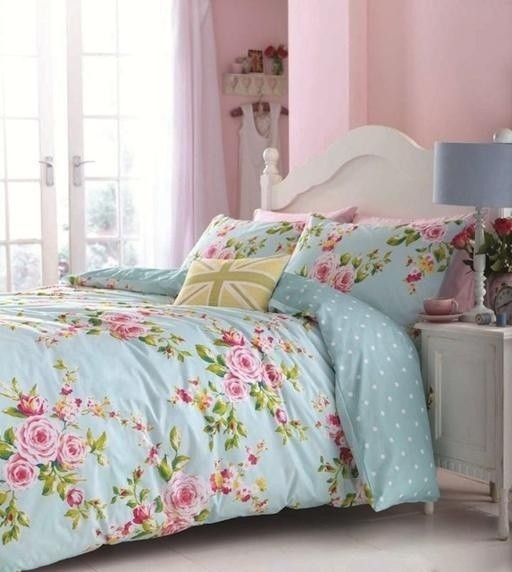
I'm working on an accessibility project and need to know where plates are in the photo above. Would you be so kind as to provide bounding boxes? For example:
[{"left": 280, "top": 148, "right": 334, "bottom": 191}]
[{"left": 416, "top": 312, "right": 463, "bottom": 322}]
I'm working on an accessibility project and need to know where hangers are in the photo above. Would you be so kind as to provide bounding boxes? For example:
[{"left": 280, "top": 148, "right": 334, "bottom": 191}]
[{"left": 229, "top": 89, "right": 289, "bottom": 117}]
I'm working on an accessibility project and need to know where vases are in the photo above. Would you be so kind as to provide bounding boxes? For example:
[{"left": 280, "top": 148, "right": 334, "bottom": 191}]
[
  {"left": 484, "top": 274, "right": 512, "bottom": 310},
  {"left": 272, "top": 56, "right": 283, "bottom": 75}
]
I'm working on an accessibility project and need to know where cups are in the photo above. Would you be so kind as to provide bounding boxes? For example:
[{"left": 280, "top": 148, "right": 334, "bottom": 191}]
[
  {"left": 232, "top": 64, "right": 243, "bottom": 74},
  {"left": 424, "top": 296, "right": 458, "bottom": 315}
]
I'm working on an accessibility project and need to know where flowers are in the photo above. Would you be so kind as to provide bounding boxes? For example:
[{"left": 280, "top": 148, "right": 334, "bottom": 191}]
[
  {"left": 264, "top": 43, "right": 288, "bottom": 72},
  {"left": 450, "top": 215, "right": 510, "bottom": 274}
]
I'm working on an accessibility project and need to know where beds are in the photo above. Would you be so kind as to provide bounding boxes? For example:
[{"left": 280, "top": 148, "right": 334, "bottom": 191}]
[{"left": 0, "top": 124, "right": 512, "bottom": 571}]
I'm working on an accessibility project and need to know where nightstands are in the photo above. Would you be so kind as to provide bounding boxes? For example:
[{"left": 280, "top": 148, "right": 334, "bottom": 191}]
[{"left": 412, "top": 323, "right": 512, "bottom": 543}]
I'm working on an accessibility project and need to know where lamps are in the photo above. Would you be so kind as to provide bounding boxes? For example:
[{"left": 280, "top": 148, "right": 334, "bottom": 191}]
[{"left": 432, "top": 141, "right": 512, "bottom": 321}]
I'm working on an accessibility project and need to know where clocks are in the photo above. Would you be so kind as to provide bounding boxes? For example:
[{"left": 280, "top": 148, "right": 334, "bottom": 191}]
[{"left": 494, "top": 284, "right": 511, "bottom": 325}]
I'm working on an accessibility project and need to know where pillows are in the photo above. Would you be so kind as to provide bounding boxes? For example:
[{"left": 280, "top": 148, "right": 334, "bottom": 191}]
[
  {"left": 282, "top": 210, "right": 466, "bottom": 331},
  {"left": 172, "top": 256, "right": 288, "bottom": 313},
  {"left": 252, "top": 207, "right": 357, "bottom": 224},
  {"left": 350, "top": 211, "right": 479, "bottom": 310},
  {"left": 180, "top": 213, "right": 304, "bottom": 273}
]
